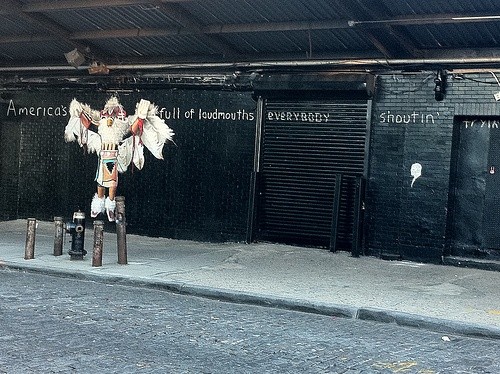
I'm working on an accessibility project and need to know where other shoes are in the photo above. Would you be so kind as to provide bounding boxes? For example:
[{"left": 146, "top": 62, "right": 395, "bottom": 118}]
[
  {"left": 106, "top": 197, "right": 117, "bottom": 222},
  {"left": 91, "top": 193, "right": 105, "bottom": 218}
]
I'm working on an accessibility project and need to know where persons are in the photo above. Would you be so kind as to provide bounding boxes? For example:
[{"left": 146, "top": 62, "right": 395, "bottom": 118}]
[{"left": 63, "top": 94, "right": 179, "bottom": 222}]
